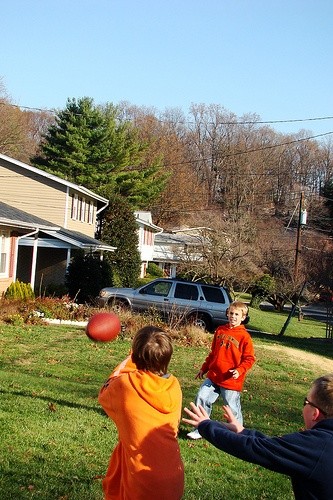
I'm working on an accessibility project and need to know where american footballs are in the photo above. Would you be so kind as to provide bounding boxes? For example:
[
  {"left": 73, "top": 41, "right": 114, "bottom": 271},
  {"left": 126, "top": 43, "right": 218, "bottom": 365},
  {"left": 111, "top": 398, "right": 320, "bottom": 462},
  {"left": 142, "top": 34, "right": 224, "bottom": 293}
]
[{"left": 84, "top": 312, "right": 123, "bottom": 344}]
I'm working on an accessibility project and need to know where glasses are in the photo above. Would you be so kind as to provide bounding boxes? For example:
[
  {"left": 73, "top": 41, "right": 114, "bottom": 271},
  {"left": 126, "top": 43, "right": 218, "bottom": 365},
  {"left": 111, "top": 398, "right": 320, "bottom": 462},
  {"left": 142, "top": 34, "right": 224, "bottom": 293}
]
[{"left": 303, "top": 397, "right": 327, "bottom": 418}]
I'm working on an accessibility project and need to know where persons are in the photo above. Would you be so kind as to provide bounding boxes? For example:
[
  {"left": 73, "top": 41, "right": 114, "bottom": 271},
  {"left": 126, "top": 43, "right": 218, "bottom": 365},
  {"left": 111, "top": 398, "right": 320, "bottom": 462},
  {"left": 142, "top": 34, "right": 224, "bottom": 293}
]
[
  {"left": 186, "top": 300, "right": 255, "bottom": 440},
  {"left": 98, "top": 325, "right": 185, "bottom": 500},
  {"left": 182, "top": 372, "right": 333, "bottom": 500}
]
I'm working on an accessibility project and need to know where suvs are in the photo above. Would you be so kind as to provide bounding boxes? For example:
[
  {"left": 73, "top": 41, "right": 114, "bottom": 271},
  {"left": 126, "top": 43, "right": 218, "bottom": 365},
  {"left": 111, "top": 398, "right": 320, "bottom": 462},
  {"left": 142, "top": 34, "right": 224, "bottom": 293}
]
[{"left": 96, "top": 278, "right": 249, "bottom": 333}]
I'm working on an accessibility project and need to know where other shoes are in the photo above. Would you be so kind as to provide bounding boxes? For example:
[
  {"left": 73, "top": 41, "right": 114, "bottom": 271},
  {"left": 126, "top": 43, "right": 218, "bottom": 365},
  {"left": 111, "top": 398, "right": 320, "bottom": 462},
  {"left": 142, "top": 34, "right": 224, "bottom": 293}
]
[{"left": 186, "top": 429, "right": 203, "bottom": 439}]
